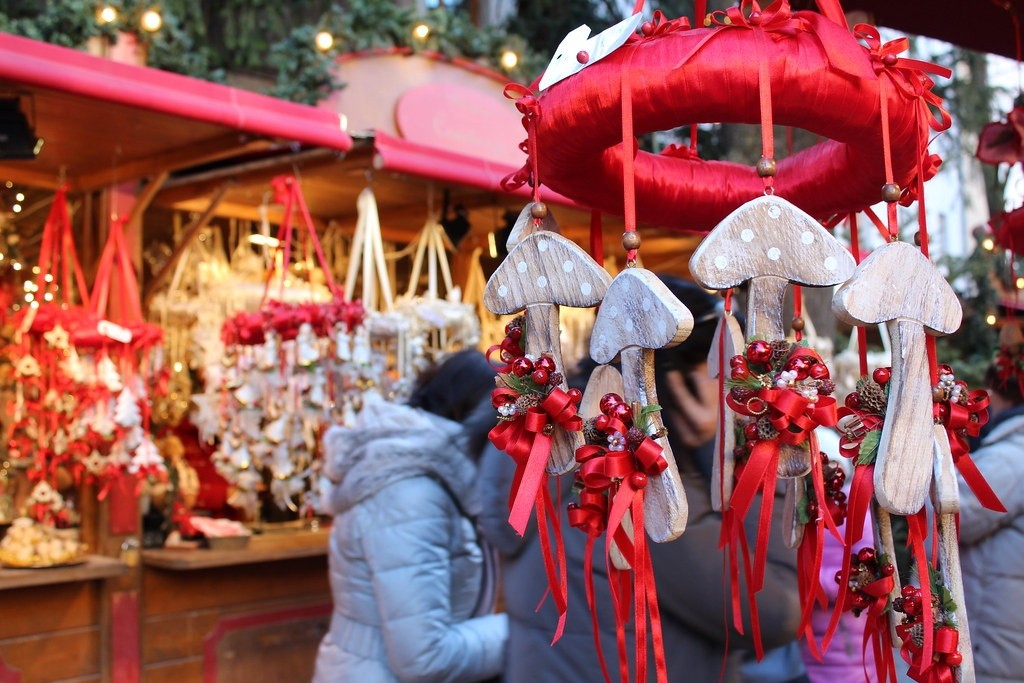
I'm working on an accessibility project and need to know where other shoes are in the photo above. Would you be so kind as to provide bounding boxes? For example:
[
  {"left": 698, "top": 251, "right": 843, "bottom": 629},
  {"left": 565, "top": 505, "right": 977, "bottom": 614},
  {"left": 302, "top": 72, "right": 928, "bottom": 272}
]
[{"left": 728, "top": 649, "right": 750, "bottom": 683}]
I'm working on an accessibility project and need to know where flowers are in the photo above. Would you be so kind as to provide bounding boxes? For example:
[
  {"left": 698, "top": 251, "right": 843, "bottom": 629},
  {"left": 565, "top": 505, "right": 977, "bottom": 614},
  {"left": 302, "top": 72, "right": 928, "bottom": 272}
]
[
  {"left": 568, "top": 392, "right": 675, "bottom": 683},
  {"left": 841, "top": 371, "right": 1007, "bottom": 683},
  {"left": 717, "top": 337, "right": 845, "bottom": 679},
  {"left": 485, "top": 360, "right": 586, "bottom": 647}
]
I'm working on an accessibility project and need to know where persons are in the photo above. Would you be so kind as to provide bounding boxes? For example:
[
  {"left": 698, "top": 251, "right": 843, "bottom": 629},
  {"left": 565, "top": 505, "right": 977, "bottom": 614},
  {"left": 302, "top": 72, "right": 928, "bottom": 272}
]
[
  {"left": 303, "top": 348, "right": 515, "bottom": 683},
  {"left": 478, "top": 272, "right": 813, "bottom": 683},
  {"left": 732, "top": 337, "right": 1023, "bottom": 683}
]
